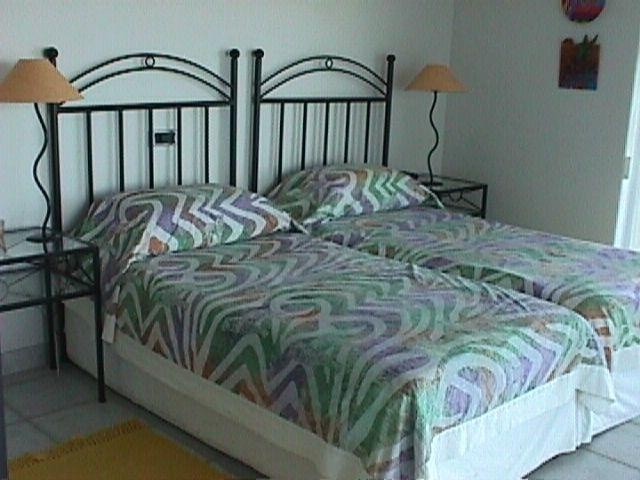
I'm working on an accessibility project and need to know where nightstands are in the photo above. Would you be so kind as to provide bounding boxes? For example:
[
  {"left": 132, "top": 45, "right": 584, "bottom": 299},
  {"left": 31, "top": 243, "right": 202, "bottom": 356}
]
[
  {"left": 0, "top": 226, "right": 104, "bottom": 404},
  {"left": 413, "top": 174, "right": 487, "bottom": 217}
]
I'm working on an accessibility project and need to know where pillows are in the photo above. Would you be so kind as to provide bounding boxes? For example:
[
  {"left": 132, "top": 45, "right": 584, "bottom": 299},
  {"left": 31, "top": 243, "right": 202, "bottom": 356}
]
[
  {"left": 265, "top": 166, "right": 429, "bottom": 222},
  {"left": 75, "top": 185, "right": 287, "bottom": 264}
]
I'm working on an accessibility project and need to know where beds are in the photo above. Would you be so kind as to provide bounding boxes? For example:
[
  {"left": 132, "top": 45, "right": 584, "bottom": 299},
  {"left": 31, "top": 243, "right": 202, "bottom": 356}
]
[{"left": 252, "top": 48, "right": 640, "bottom": 475}]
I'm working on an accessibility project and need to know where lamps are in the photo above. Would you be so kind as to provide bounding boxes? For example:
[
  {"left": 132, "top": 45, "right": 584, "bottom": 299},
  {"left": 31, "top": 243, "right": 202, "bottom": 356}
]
[
  {"left": 45, "top": 49, "right": 615, "bottom": 449},
  {"left": 404, "top": 63, "right": 468, "bottom": 185},
  {"left": 1, "top": 59, "right": 83, "bottom": 242}
]
